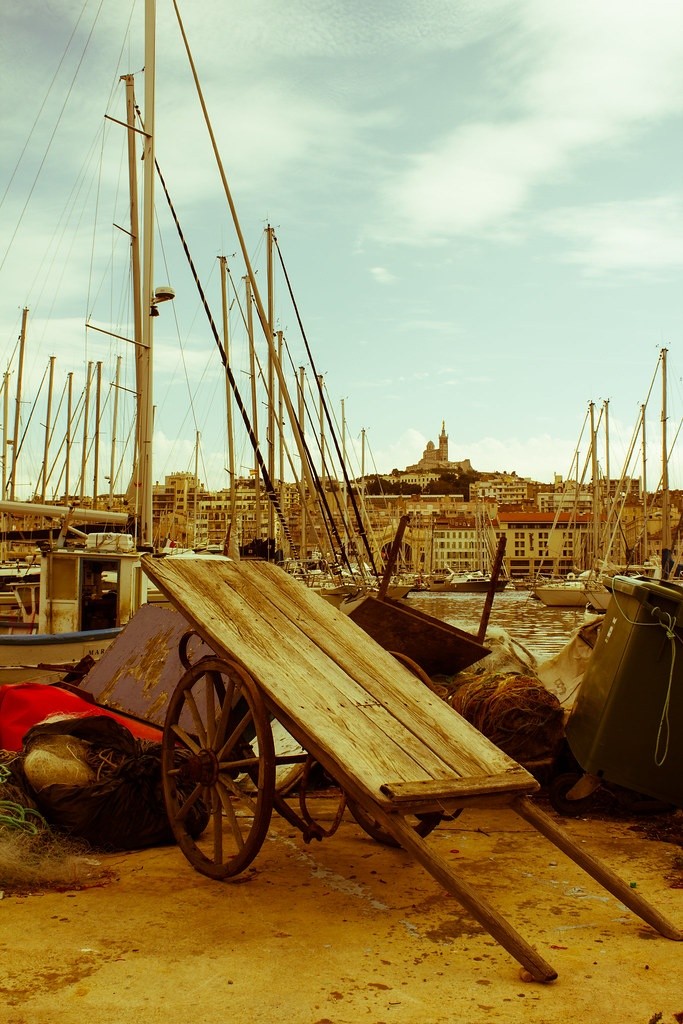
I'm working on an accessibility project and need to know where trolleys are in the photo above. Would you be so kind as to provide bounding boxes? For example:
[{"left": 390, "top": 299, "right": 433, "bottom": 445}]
[{"left": 140, "top": 554, "right": 683, "bottom": 983}]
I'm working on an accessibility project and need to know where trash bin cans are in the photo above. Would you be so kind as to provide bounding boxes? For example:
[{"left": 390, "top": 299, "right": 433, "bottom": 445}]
[{"left": 566, "top": 574, "right": 683, "bottom": 809}]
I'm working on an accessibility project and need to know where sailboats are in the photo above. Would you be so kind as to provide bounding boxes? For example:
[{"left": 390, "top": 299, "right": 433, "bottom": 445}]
[
  {"left": 0, "top": 0, "right": 509, "bottom": 686},
  {"left": 512, "top": 348, "right": 683, "bottom": 611}
]
